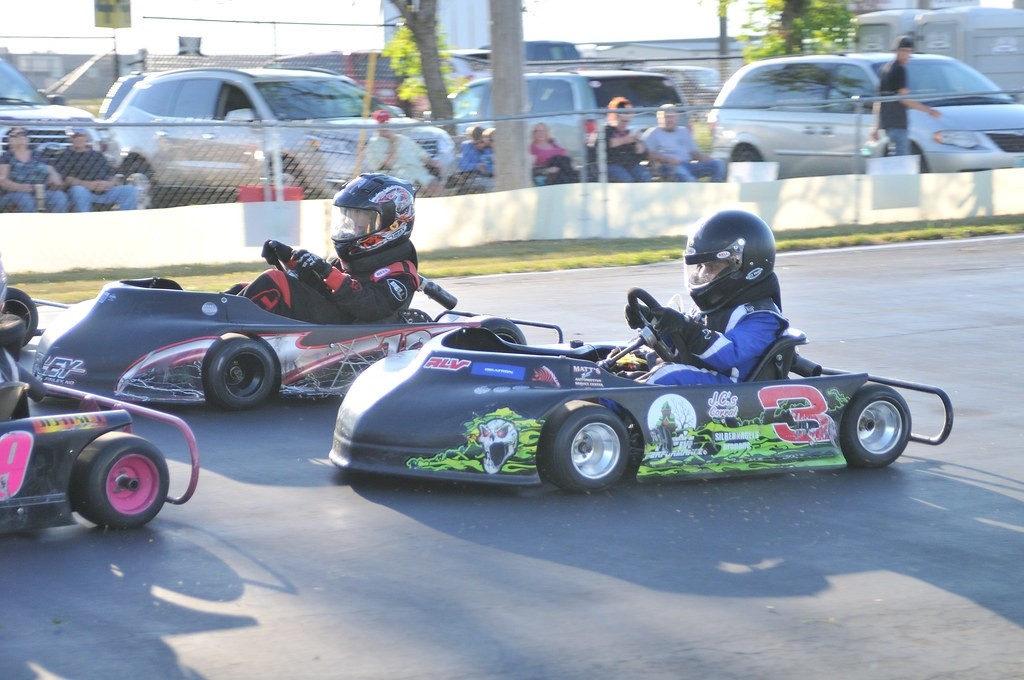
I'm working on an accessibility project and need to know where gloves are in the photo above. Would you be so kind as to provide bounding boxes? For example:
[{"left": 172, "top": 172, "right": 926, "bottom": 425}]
[
  {"left": 626, "top": 305, "right": 656, "bottom": 328},
  {"left": 654, "top": 309, "right": 699, "bottom": 342},
  {"left": 291, "top": 250, "right": 330, "bottom": 280},
  {"left": 261, "top": 240, "right": 296, "bottom": 266}
]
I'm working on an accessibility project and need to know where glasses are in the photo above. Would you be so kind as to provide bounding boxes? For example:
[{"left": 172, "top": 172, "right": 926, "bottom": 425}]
[{"left": 8, "top": 131, "right": 24, "bottom": 138}]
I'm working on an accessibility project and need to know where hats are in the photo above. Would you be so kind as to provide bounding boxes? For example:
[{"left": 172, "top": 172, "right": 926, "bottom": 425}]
[
  {"left": 67, "top": 126, "right": 86, "bottom": 135},
  {"left": 897, "top": 37, "right": 917, "bottom": 50}
]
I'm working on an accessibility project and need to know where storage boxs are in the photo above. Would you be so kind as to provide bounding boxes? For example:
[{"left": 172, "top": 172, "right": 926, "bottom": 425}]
[{"left": 237, "top": 186, "right": 303, "bottom": 202}]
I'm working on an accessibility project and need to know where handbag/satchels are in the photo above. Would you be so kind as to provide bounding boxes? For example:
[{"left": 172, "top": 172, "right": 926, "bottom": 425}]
[{"left": 863, "top": 140, "right": 894, "bottom": 164}]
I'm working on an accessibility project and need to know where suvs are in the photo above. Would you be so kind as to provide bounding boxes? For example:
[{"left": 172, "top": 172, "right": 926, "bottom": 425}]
[
  {"left": 105, "top": 68, "right": 455, "bottom": 199},
  {"left": 0, "top": 57, "right": 101, "bottom": 160}
]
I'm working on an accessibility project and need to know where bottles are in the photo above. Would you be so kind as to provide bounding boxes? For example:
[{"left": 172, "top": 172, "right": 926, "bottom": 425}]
[{"left": 862, "top": 136, "right": 879, "bottom": 155}]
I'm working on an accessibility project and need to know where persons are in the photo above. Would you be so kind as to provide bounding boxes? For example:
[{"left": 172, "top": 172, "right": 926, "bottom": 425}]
[
  {"left": 588, "top": 96, "right": 651, "bottom": 182},
  {"left": 367, "top": 110, "right": 445, "bottom": 197},
  {"left": 529, "top": 122, "right": 567, "bottom": 186},
  {"left": 871, "top": 36, "right": 941, "bottom": 155},
  {"left": 225, "top": 173, "right": 419, "bottom": 325},
  {"left": 0, "top": 128, "right": 67, "bottom": 213},
  {"left": 56, "top": 131, "right": 137, "bottom": 212},
  {"left": 643, "top": 104, "right": 724, "bottom": 182},
  {"left": 458, "top": 125, "right": 494, "bottom": 175},
  {"left": 597, "top": 211, "right": 789, "bottom": 385}
]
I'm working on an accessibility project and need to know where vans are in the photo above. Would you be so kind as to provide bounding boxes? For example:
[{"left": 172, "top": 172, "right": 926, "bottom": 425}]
[
  {"left": 707, "top": 52, "right": 1024, "bottom": 179},
  {"left": 448, "top": 70, "right": 691, "bottom": 169}
]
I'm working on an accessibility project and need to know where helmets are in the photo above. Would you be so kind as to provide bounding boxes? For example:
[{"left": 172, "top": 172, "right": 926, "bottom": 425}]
[
  {"left": 332, "top": 172, "right": 414, "bottom": 269},
  {"left": 684, "top": 210, "right": 775, "bottom": 315}
]
